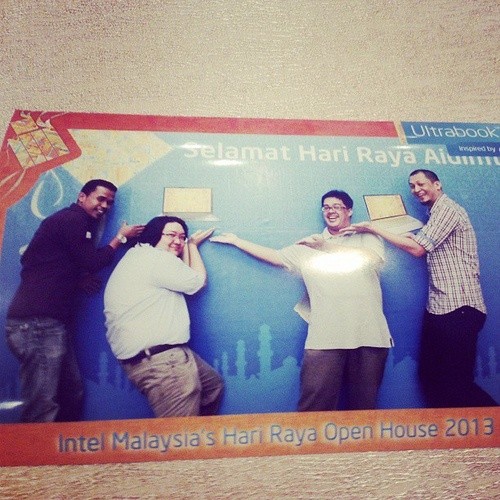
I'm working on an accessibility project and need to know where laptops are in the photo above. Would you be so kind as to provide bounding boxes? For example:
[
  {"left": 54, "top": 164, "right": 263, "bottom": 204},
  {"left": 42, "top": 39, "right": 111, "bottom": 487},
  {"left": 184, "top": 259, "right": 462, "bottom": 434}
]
[
  {"left": 363, "top": 194, "right": 423, "bottom": 236},
  {"left": 163, "top": 187, "right": 222, "bottom": 222}
]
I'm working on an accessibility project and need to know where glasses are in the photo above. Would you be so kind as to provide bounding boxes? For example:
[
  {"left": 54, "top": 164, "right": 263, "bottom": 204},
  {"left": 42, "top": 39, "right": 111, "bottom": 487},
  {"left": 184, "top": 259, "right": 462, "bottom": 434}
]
[
  {"left": 322, "top": 205, "right": 349, "bottom": 213},
  {"left": 158, "top": 230, "right": 187, "bottom": 242}
]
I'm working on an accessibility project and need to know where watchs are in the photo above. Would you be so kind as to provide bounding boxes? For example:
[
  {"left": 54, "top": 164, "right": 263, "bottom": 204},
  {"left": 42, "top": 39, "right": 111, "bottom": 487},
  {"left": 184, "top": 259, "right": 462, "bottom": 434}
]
[{"left": 116, "top": 234, "right": 127, "bottom": 244}]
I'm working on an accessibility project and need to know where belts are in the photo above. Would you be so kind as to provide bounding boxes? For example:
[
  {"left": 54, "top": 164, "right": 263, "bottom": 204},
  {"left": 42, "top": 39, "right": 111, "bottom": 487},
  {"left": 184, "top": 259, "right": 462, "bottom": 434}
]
[{"left": 123, "top": 343, "right": 186, "bottom": 364}]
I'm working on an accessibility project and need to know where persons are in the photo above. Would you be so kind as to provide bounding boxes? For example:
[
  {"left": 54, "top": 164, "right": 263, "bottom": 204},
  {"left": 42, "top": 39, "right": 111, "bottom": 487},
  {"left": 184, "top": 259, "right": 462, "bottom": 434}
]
[
  {"left": 339, "top": 169, "right": 498, "bottom": 409},
  {"left": 104, "top": 216, "right": 224, "bottom": 418},
  {"left": 209, "top": 190, "right": 395, "bottom": 412},
  {"left": 6, "top": 179, "right": 145, "bottom": 423}
]
